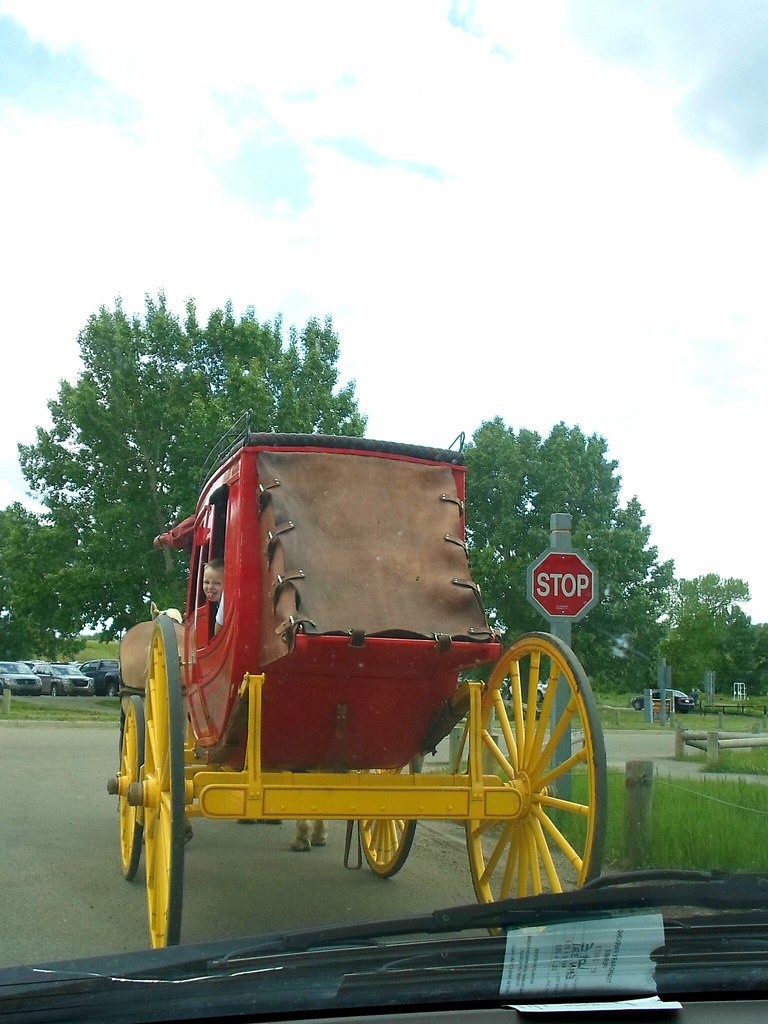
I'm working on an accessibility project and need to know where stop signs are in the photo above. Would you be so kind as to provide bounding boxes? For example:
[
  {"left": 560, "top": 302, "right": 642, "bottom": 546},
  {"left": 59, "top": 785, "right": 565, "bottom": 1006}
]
[{"left": 526, "top": 548, "right": 600, "bottom": 623}]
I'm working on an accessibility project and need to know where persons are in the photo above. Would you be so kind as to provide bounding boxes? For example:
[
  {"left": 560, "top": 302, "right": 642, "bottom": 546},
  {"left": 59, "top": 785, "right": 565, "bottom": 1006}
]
[{"left": 203, "top": 558, "right": 224, "bottom": 635}]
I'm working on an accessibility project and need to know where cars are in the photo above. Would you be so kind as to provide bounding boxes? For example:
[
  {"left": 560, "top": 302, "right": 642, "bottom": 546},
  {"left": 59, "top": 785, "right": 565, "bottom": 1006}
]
[
  {"left": 79, "top": 659, "right": 120, "bottom": 697},
  {"left": 631, "top": 689, "right": 696, "bottom": 713},
  {"left": 0, "top": 662, "right": 43, "bottom": 696},
  {"left": 32, "top": 664, "right": 95, "bottom": 697}
]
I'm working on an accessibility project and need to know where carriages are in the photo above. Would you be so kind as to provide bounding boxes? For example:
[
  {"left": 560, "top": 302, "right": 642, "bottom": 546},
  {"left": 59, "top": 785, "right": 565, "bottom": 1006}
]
[{"left": 108, "top": 412, "right": 606, "bottom": 951}]
